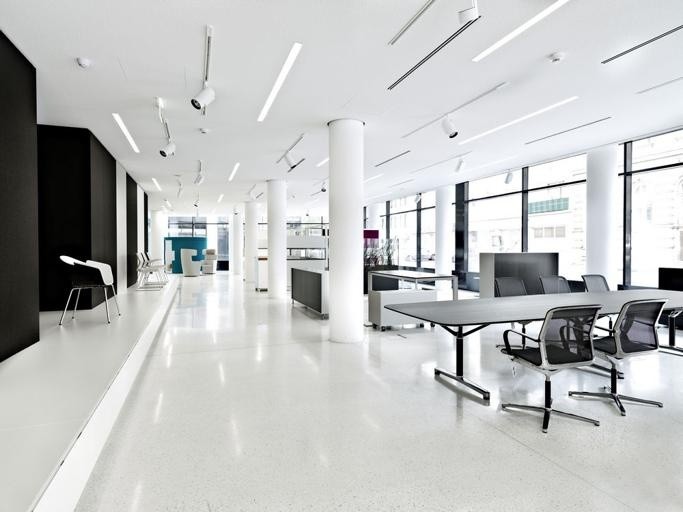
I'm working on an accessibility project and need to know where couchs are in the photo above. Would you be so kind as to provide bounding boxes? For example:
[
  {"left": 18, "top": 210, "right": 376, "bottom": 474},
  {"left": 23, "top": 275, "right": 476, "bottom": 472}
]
[
  {"left": 289, "top": 266, "right": 329, "bottom": 320},
  {"left": 367, "top": 288, "right": 438, "bottom": 331}
]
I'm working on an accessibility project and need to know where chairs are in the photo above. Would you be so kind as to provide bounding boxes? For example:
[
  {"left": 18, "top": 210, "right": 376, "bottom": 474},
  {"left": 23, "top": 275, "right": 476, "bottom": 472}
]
[
  {"left": 581, "top": 273, "right": 611, "bottom": 338},
  {"left": 57, "top": 254, "right": 122, "bottom": 326},
  {"left": 538, "top": 274, "right": 578, "bottom": 347},
  {"left": 494, "top": 275, "right": 535, "bottom": 350},
  {"left": 134, "top": 251, "right": 169, "bottom": 293},
  {"left": 499, "top": 301, "right": 604, "bottom": 433},
  {"left": 179, "top": 248, "right": 201, "bottom": 277},
  {"left": 567, "top": 297, "right": 669, "bottom": 418},
  {"left": 200, "top": 248, "right": 218, "bottom": 274},
  {"left": 624, "top": 267, "right": 683, "bottom": 350}
]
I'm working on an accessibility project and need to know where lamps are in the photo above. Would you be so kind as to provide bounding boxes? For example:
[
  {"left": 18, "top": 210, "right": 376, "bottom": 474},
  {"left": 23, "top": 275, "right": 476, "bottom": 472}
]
[
  {"left": 414, "top": 192, "right": 423, "bottom": 204},
  {"left": 504, "top": 172, "right": 513, "bottom": 185},
  {"left": 176, "top": 171, "right": 203, "bottom": 208},
  {"left": 159, "top": 138, "right": 177, "bottom": 159},
  {"left": 438, "top": 115, "right": 459, "bottom": 140},
  {"left": 190, "top": 80, "right": 216, "bottom": 111},
  {"left": 320, "top": 180, "right": 328, "bottom": 193}
]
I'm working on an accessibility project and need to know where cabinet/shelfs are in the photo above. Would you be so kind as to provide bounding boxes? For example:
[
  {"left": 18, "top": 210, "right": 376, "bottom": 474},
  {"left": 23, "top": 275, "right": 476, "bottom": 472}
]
[
  {"left": 363, "top": 237, "right": 400, "bottom": 292},
  {"left": 255, "top": 234, "right": 327, "bottom": 295},
  {"left": 479, "top": 251, "right": 560, "bottom": 297}
]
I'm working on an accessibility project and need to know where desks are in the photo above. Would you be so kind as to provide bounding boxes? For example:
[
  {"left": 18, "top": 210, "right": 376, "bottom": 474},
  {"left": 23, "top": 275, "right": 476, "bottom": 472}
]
[
  {"left": 382, "top": 283, "right": 683, "bottom": 402},
  {"left": 366, "top": 269, "right": 459, "bottom": 303}
]
